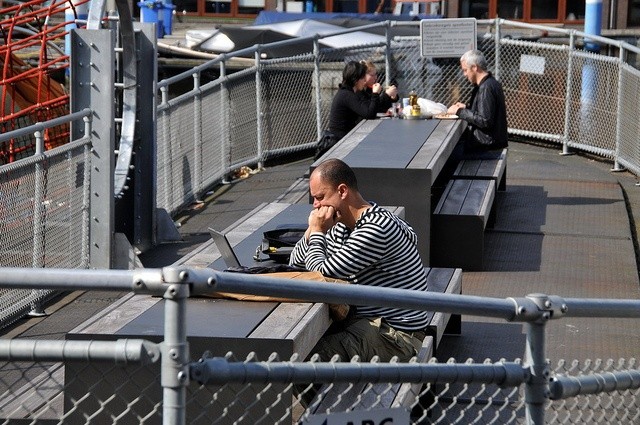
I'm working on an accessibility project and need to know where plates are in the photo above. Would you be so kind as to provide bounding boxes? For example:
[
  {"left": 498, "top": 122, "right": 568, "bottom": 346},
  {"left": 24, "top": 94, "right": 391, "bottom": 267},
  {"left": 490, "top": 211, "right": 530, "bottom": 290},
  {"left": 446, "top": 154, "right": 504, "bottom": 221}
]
[{"left": 433, "top": 114, "right": 459, "bottom": 120}]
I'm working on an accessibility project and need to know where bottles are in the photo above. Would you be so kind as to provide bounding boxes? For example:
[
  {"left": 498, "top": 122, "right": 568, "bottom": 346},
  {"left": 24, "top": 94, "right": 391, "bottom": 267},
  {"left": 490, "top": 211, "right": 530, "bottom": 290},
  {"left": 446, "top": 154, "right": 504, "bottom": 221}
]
[{"left": 409, "top": 88, "right": 417, "bottom": 106}]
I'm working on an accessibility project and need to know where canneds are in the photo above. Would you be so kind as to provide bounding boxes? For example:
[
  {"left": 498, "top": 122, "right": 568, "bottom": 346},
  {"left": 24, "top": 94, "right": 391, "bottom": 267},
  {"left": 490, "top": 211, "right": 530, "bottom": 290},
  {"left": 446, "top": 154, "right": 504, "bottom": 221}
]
[{"left": 412, "top": 105, "right": 420, "bottom": 120}]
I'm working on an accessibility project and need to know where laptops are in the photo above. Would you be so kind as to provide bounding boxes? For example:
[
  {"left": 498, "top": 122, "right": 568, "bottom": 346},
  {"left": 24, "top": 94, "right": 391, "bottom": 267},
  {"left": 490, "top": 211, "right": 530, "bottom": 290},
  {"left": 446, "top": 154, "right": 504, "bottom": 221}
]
[{"left": 207, "top": 225, "right": 273, "bottom": 275}]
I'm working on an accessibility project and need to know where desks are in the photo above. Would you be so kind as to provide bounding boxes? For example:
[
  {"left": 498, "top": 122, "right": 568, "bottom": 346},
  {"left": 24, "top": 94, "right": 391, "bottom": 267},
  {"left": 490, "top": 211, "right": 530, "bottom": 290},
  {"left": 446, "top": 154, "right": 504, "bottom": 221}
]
[
  {"left": 60, "top": 200, "right": 408, "bottom": 425},
  {"left": 307, "top": 111, "right": 468, "bottom": 267}
]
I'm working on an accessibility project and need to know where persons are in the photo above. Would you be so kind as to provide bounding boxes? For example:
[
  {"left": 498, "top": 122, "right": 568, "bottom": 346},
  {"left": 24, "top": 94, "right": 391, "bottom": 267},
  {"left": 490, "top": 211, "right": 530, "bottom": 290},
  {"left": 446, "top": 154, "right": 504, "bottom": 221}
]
[
  {"left": 359, "top": 57, "right": 400, "bottom": 113},
  {"left": 447, "top": 48, "right": 508, "bottom": 173},
  {"left": 312, "top": 59, "right": 384, "bottom": 163},
  {"left": 289, "top": 158, "right": 430, "bottom": 424}
]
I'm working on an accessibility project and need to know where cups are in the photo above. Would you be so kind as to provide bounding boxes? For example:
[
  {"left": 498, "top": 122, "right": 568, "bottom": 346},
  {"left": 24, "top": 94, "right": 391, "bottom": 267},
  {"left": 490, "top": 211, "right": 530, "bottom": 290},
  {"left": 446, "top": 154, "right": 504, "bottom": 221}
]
[
  {"left": 410, "top": 104, "right": 420, "bottom": 116},
  {"left": 392, "top": 102, "right": 400, "bottom": 119},
  {"left": 403, "top": 98, "right": 410, "bottom": 108}
]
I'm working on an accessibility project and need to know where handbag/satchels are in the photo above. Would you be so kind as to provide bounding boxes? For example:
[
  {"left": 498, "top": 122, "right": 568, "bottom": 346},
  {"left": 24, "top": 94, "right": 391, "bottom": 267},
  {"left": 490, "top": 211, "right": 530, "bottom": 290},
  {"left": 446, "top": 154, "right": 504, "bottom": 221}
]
[
  {"left": 252, "top": 223, "right": 310, "bottom": 262},
  {"left": 312, "top": 131, "right": 337, "bottom": 162}
]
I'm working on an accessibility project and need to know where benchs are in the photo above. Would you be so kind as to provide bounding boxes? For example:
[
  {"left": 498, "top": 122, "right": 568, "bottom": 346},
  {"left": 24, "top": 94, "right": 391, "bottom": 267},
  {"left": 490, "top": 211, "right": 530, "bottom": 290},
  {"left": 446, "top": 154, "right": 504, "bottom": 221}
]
[
  {"left": 1, "top": 361, "right": 64, "bottom": 424},
  {"left": 298, "top": 329, "right": 436, "bottom": 425},
  {"left": 451, "top": 145, "right": 508, "bottom": 193},
  {"left": 431, "top": 177, "right": 498, "bottom": 272},
  {"left": 273, "top": 177, "right": 309, "bottom": 203},
  {"left": 423, "top": 265, "right": 463, "bottom": 350}
]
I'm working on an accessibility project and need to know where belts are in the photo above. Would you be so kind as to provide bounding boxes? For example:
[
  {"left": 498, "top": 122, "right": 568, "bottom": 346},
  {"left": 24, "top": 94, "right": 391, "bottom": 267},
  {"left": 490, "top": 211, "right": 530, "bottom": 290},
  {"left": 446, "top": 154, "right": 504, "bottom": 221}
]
[{"left": 404, "top": 326, "right": 426, "bottom": 343}]
